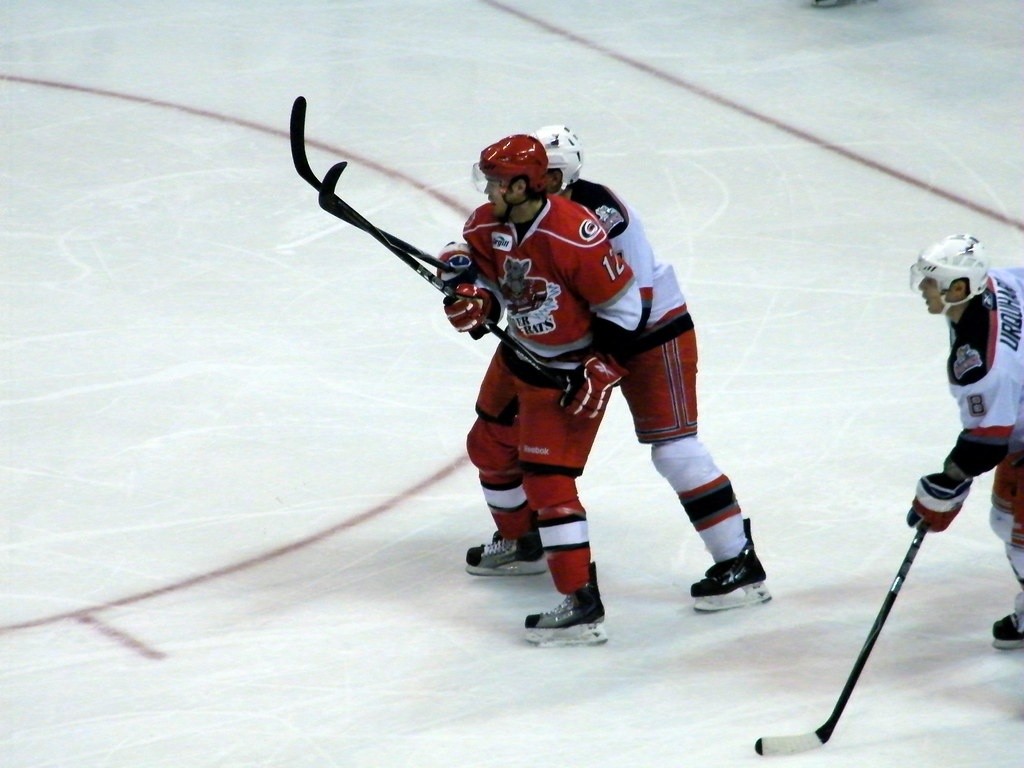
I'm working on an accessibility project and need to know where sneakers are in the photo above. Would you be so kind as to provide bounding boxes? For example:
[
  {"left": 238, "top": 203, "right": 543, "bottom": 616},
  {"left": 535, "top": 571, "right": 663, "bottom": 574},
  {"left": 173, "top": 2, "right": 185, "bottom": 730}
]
[
  {"left": 521, "top": 561, "right": 609, "bottom": 648},
  {"left": 991, "top": 611, "right": 1024, "bottom": 648},
  {"left": 690, "top": 518, "right": 772, "bottom": 611},
  {"left": 464, "top": 511, "right": 549, "bottom": 576}
]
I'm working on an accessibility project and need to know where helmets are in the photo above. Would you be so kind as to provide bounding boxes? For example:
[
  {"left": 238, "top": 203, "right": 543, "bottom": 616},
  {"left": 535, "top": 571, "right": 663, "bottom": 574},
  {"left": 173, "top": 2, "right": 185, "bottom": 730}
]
[
  {"left": 478, "top": 134, "right": 549, "bottom": 180},
  {"left": 916, "top": 233, "right": 989, "bottom": 295},
  {"left": 530, "top": 125, "right": 584, "bottom": 190}
]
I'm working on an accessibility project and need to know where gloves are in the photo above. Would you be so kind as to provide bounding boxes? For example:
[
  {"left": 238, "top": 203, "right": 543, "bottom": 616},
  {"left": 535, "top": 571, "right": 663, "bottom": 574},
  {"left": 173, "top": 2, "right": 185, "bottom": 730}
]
[
  {"left": 435, "top": 240, "right": 479, "bottom": 288},
  {"left": 905, "top": 473, "right": 974, "bottom": 533},
  {"left": 443, "top": 283, "right": 501, "bottom": 340},
  {"left": 557, "top": 351, "right": 630, "bottom": 419}
]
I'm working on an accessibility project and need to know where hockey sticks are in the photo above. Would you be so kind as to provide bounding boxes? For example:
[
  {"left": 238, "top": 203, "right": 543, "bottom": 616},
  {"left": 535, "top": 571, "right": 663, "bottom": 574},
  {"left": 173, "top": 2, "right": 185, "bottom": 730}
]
[
  {"left": 752, "top": 527, "right": 928, "bottom": 758},
  {"left": 288, "top": 93, "right": 569, "bottom": 390},
  {"left": 316, "top": 158, "right": 455, "bottom": 274}
]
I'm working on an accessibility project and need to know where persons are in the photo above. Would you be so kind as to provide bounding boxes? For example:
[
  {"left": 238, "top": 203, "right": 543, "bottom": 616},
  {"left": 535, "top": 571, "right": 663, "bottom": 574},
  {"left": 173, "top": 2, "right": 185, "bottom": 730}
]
[
  {"left": 906, "top": 233, "right": 1024, "bottom": 642},
  {"left": 437, "top": 125, "right": 767, "bottom": 598},
  {"left": 442, "top": 134, "right": 642, "bottom": 629}
]
